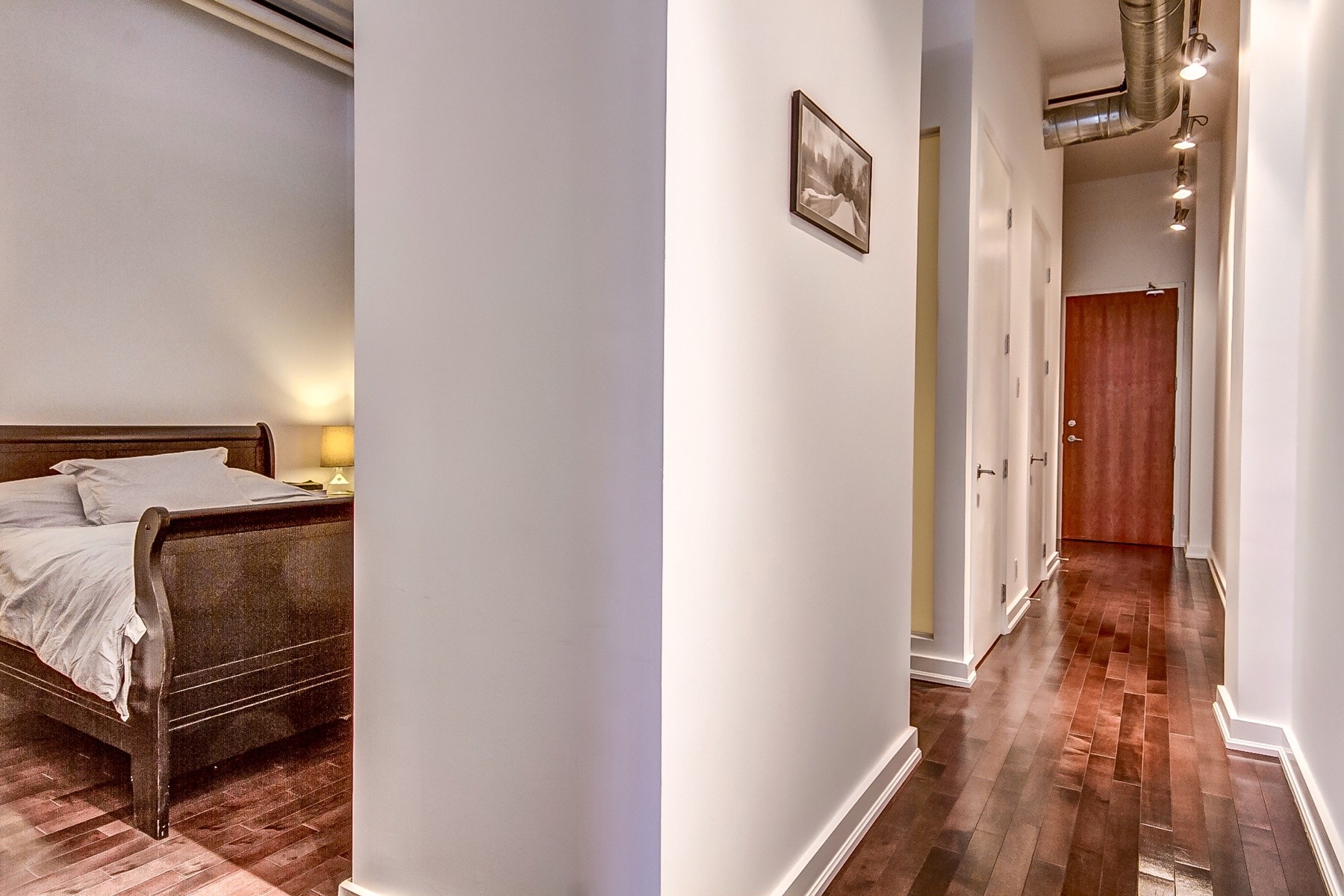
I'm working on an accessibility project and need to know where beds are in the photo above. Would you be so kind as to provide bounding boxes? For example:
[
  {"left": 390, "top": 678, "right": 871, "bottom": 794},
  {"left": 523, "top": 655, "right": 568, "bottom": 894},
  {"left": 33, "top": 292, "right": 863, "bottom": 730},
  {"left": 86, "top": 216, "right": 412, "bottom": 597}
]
[{"left": 0, "top": 422, "right": 353, "bottom": 838}]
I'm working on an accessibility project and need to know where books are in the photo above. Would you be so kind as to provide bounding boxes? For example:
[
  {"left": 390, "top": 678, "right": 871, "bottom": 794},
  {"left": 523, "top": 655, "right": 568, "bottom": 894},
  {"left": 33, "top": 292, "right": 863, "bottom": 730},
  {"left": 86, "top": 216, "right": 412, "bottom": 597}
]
[
  {"left": 308, "top": 490, "right": 328, "bottom": 497},
  {"left": 283, "top": 480, "right": 323, "bottom": 490}
]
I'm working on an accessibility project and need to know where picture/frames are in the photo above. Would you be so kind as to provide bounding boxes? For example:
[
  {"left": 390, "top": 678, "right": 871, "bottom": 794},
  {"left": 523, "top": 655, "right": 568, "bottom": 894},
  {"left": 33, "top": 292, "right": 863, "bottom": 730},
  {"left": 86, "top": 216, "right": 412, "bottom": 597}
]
[{"left": 790, "top": 90, "right": 872, "bottom": 254}]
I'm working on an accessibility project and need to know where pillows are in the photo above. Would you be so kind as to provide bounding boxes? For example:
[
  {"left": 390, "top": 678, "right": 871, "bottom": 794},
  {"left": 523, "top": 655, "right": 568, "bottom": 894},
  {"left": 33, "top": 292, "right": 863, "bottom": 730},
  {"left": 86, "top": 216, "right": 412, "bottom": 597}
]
[{"left": 49, "top": 446, "right": 250, "bottom": 528}]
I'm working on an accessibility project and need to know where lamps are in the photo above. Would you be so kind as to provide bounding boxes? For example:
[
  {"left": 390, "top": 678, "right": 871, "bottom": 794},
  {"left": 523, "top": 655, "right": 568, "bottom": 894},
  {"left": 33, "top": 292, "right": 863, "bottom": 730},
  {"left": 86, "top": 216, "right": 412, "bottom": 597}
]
[
  {"left": 1171, "top": 169, "right": 1195, "bottom": 199},
  {"left": 319, "top": 424, "right": 355, "bottom": 496},
  {"left": 1180, "top": 33, "right": 1216, "bottom": 81},
  {"left": 1169, "top": 208, "right": 1190, "bottom": 231},
  {"left": 1173, "top": 114, "right": 1208, "bottom": 149}
]
